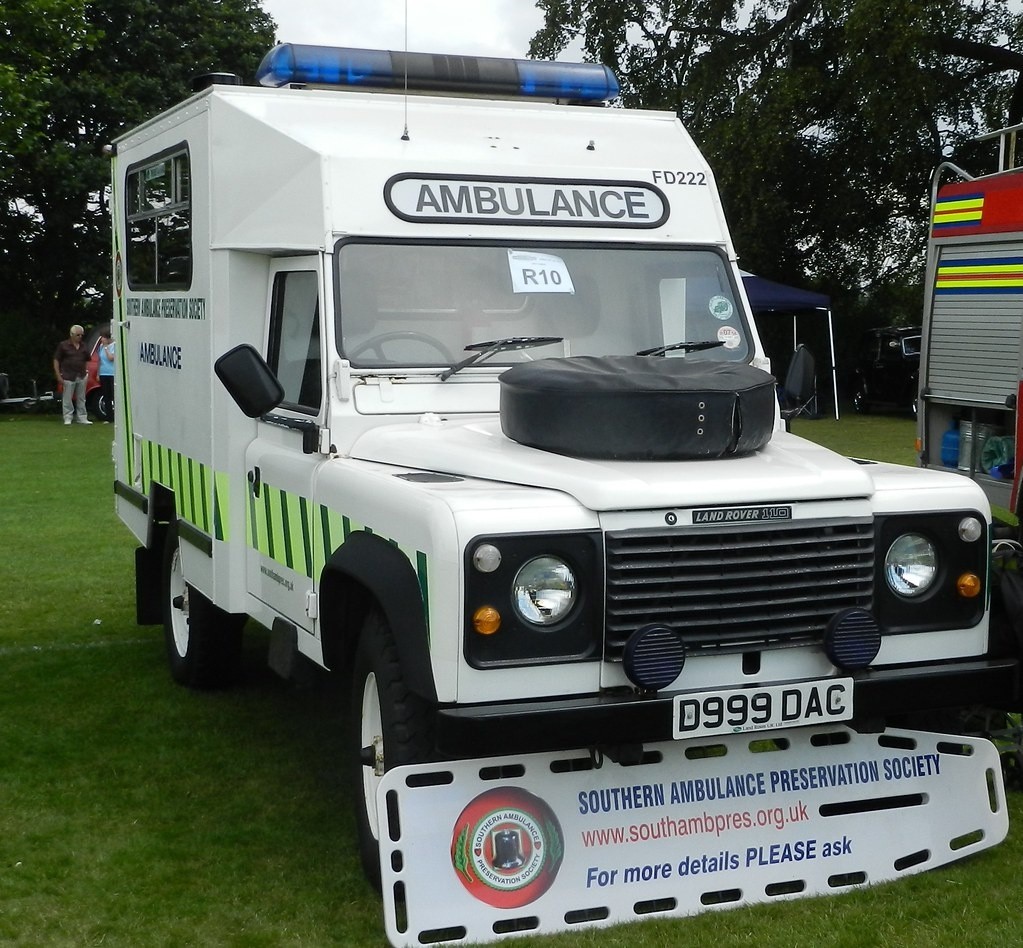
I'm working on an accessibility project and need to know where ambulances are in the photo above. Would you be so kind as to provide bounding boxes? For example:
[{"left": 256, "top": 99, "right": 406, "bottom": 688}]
[{"left": 102, "top": 39, "right": 1023, "bottom": 886}]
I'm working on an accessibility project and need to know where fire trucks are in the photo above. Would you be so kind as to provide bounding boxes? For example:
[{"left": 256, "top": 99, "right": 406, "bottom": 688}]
[{"left": 911, "top": 121, "right": 1023, "bottom": 547}]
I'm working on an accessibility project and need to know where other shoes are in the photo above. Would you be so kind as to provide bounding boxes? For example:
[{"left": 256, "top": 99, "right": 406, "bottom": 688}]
[
  {"left": 103, "top": 420, "right": 109, "bottom": 425},
  {"left": 77, "top": 419, "right": 93, "bottom": 425},
  {"left": 64, "top": 420, "right": 71, "bottom": 425}
]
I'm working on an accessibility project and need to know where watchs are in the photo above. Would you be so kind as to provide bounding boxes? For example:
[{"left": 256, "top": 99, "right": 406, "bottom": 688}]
[{"left": 105, "top": 347, "right": 107, "bottom": 350}]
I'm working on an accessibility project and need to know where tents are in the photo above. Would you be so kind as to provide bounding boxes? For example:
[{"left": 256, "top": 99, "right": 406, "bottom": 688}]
[{"left": 739, "top": 269, "right": 839, "bottom": 421}]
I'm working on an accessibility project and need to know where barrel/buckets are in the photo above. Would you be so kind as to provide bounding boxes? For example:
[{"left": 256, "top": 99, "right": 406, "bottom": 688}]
[{"left": 958, "top": 420, "right": 997, "bottom": 472}]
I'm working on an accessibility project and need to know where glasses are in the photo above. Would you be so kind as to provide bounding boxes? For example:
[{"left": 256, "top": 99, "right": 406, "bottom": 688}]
[{"left": 72, "top": 332, "right": 84, "bottom": 337}]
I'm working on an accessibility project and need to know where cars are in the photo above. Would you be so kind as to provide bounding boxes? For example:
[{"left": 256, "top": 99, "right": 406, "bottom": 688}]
[
  {"left": 820, "top": 324, "right": 924, "bottom": 422},
  {"left": 53, "top": 321, "right": 115, "bottom": 422}
]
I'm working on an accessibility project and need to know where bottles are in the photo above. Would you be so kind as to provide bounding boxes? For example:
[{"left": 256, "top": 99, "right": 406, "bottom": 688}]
[
  {"left": 979, "top": 435, "right": 1016, "bottom": 476},
  {"left": 940, "top": 419, "right": 963, "bottom": 468}
]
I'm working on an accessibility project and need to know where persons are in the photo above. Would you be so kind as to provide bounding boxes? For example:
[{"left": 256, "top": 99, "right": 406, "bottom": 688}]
[
  {"left": 53, "top": 324, "right": 93, "bottom": 425},
  {"left": 96, "top": 330, "right": 115, "bottom": 424}
]
